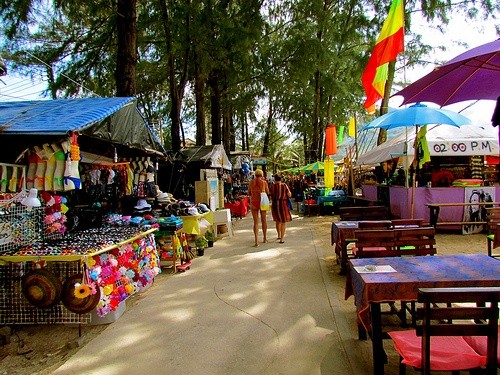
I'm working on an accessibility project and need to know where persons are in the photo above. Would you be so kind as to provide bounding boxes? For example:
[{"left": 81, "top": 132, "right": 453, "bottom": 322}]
[
  {"left": 247, "top": 169, "right": 272, "bottom": 249},
  {"left": 269, "top": 174, "right": 293, "bottom": 243}
]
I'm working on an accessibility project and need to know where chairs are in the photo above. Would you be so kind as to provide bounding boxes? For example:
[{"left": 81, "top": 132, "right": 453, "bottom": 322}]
[
  {"left": 304, "top": 194, "right": 321, "bottom": 217},
  {"left": 339, "top": 206, "right": 500, "bottom": 375}
]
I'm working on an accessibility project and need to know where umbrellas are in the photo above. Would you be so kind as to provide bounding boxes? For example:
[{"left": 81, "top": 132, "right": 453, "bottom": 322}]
[
  {"left": 357, "top": 104, "right": 473, "bottom": 220},
  {"left": 279, "top": 161, "right": 340, "bottom": 177},
  {"left": 388, "top": 38, "right": 500, "bottom": 158}
]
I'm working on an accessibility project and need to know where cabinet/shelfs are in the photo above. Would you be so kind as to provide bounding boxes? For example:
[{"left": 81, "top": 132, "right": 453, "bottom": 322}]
[
  {"left": 195, "top": 181, "right": 224, "bottom": 211},
  {"left": 160, "top": 228, "right": 185, "bottom": 273}
]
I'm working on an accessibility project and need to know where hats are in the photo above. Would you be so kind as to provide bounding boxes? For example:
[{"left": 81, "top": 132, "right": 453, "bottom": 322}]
[
  {"left": 254, "top": 169, "right": 263, "bottom": 176},
  {"left": 162, "top": 199, "right": 209, "bottom": 216},
  {"left": 21, "top": 267, "right": 64, "bottom": 309},
  {"left": 61, "top": 275, "right": 100, "bottom": 314},
  {"left": 133, "top": 199, "right": 152, "bottom": 214}
]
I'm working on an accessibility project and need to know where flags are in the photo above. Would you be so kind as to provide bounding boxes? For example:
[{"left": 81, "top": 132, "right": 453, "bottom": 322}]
[{"left": 357, "top": 0, "right": 405, "bottom": 109}]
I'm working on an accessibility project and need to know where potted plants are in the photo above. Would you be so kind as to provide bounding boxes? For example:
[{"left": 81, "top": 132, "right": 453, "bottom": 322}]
[
  {"left": 205, "top": 229, "right": 214, "bottom": 247},
  {"left": 195, "top": 237, "right": 205, "bottom": 256}
]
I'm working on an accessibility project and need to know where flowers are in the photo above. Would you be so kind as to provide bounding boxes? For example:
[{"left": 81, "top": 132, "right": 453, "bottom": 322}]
[
  {"left": 72, "top": 239, "right": 160, "bottom": 319},
  {"left": 46, "top": 195, "right": 69, "bottom": 236}
]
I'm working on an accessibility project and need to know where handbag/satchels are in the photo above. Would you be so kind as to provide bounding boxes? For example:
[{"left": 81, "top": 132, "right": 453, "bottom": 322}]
[
  {"left": 288, "top": 198, "right": 293, "bottom": 211},
  {"left": 260, "top": 191, "right": 270, "bottom": 212}
]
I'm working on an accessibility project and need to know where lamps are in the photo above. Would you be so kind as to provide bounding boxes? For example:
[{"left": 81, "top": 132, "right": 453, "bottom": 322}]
[{"left": 0, "top": 187, "right": 42, "bottom": 212}]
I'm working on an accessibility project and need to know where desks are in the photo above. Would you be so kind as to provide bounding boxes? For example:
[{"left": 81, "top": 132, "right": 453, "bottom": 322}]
[
  {"left": 224, "top": 197, "right": 248, "bottom": 219},
  {"left": 347, "top": 196, "right": 381, "bottom": 207},
  {"left": 331, "top": 219, "right": 421, "bottom": 276},
  {"left": 162, "top": 209, "right": 214, "bottom": 255},
  {"left": 345, "top": 253, "right": 489, "bottom": 375},
  {"left": 424, "top": 202, "right": 500, "bottom": 239},
  {"left": 317, "top": 195, "right": 347, "bottom": 216}
]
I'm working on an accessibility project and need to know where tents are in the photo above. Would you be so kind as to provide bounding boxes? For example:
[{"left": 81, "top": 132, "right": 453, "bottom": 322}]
[{"left": 355, "top": 123, "right": 500, "bottom": 187}]
